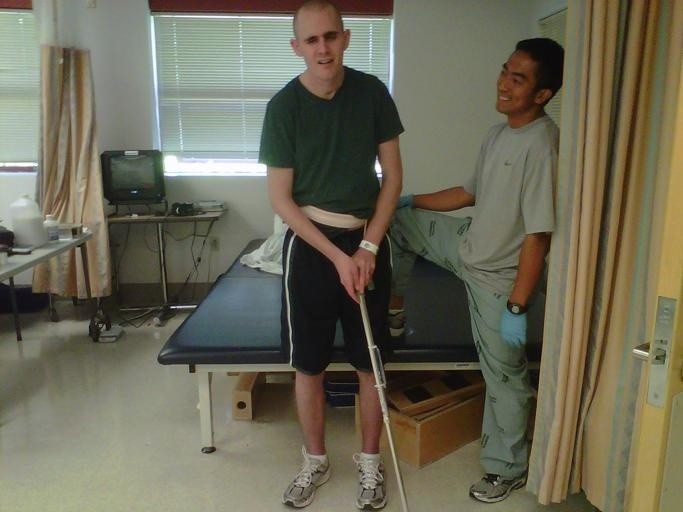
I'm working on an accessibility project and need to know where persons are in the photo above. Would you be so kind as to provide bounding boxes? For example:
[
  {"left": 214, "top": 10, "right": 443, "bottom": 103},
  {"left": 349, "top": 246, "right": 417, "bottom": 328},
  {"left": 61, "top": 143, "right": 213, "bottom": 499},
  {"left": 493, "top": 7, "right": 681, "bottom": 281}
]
[
  {"left": 257, "top": 2, "right": 406, "bottom": 510},
  {"left": 395, "top": 37, "right": 567, "bottom": 502}
]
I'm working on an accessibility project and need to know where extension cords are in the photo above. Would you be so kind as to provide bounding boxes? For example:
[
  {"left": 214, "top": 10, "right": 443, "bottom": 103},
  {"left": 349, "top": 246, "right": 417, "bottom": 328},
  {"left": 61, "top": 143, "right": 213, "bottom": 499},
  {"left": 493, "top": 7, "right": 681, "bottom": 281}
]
[{"left": 98, "top": 324, "right": 122, "bottom": 343}]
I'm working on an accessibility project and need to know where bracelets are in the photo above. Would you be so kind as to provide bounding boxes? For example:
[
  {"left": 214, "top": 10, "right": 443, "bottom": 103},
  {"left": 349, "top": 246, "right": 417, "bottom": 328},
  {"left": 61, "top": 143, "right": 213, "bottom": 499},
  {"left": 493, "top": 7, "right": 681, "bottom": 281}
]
[{"left": 358, "top": 239, "right": 379, "bottom": 255}]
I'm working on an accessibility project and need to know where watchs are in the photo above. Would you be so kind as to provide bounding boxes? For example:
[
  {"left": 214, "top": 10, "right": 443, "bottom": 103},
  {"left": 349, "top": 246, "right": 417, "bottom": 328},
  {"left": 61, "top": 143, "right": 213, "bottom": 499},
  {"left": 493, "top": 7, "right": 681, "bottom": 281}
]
[{"left": 507, "top": 299, "right": 530, "bottom": 315}]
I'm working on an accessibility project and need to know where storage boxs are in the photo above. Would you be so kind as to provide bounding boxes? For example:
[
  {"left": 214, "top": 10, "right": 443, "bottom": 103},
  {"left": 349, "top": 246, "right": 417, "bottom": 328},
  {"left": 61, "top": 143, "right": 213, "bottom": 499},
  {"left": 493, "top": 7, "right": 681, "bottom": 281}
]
[{"left": 349, "top": 385, "right": 485, "bottom": 471}]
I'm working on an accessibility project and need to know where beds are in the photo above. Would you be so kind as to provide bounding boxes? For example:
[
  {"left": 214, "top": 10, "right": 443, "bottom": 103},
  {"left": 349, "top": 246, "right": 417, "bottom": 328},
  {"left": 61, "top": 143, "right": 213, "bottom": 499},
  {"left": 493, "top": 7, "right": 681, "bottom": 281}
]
[{"left": 158, "top": 237, "right": 546, "bottom": 453}]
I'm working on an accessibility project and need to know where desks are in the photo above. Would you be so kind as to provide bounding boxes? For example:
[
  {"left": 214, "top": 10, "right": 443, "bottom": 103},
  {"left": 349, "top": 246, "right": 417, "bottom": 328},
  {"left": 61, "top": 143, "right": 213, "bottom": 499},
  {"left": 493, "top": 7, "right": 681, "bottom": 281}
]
[
  {"left": 99, "top": 209, "right": 224, "bottom": 314},
  {"left": 0, "top": 221, "right": 94, "bottom": 357}
]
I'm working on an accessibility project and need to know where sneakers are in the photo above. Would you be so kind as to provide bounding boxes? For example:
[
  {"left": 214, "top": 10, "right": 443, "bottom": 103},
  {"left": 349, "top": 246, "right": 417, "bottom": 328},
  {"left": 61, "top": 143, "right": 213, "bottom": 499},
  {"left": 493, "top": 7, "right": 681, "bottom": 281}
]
[
  {"left": 281, "top": 443, "right": 332, "bottom": 509},
  {"left": 350, "top": 452, "right": 387, "bottom": 511},
  {"left": 470, "top": 473, "right": 527, "bottom": 505},
  {"left": 385, "top": 308, "right": 408, "bottom": 337}
]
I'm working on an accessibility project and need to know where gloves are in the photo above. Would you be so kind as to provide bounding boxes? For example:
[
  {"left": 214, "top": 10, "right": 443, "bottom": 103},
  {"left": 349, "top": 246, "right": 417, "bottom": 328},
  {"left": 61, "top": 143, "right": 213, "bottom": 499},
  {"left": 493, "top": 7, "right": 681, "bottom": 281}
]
[
  {"left": 395, "top": 194, "right": 416, "bottom": 211},
  {"left": 500, "top": 306, "right": 528, "bottom": 350}
]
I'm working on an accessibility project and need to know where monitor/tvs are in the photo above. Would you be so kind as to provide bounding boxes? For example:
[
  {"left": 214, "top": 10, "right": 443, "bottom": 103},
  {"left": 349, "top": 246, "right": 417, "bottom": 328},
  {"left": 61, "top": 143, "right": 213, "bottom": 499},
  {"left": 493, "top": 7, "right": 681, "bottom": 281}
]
[{"left": 100, "top": 150, "right": 165, "bottom": 206}]
